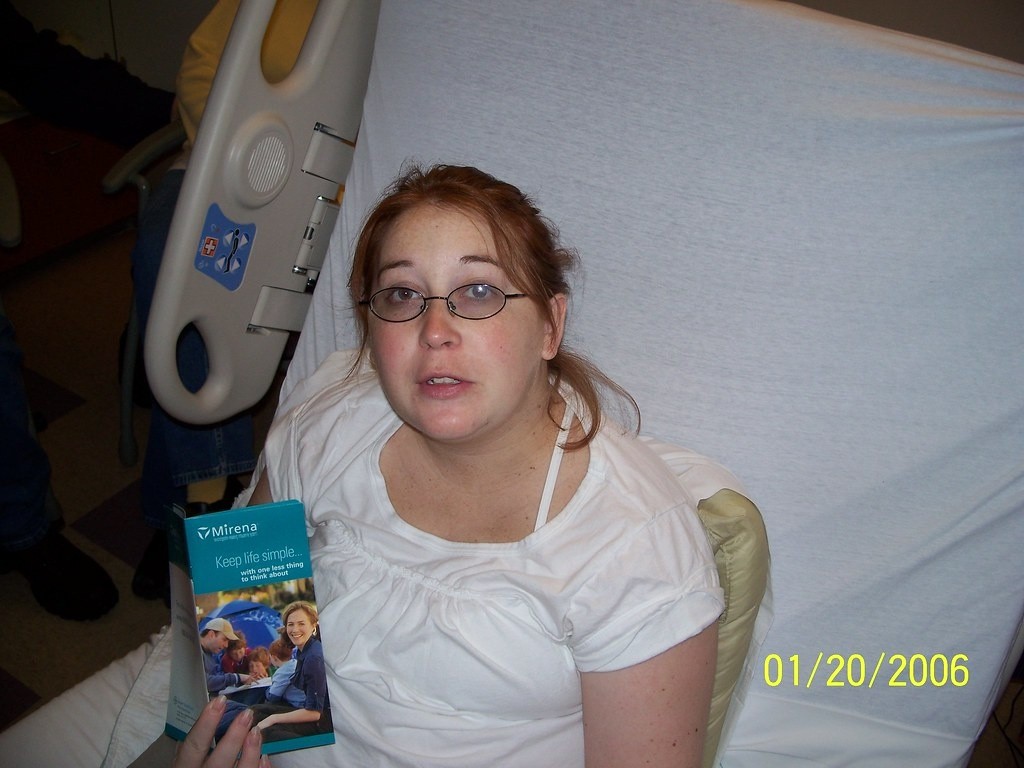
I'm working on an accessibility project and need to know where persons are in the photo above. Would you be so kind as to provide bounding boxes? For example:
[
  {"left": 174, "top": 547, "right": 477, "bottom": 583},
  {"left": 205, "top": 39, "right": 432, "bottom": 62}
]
[
  {"left": 0, "top": 297, "right": 119, "bottom": 622},
  {"left": 132, "top": 0, "right": 323, "bottom": 609},
  {"left": 173, "top": 166, "right": 724, "bottom": 768},
  {"left": 200, "top": 601, "right": 334, "bottom": 744}
]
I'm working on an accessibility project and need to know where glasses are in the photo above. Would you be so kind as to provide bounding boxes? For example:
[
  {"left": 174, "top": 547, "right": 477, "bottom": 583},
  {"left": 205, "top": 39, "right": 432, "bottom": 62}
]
[{"left": 357, "top": 282, "right": 529, "bottom": 323}]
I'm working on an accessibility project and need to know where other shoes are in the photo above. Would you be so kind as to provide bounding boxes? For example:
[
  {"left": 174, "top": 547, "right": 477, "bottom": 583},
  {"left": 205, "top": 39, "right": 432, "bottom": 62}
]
[
  {"left": 0, "top": 511, "right": 120, "bottom": 629},
  {"left": 129, "top": 490, "right": 238, "bottom": 606}
]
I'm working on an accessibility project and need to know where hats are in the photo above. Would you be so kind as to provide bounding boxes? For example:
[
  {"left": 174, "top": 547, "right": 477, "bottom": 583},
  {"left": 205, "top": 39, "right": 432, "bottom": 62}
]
[{"left": 204, "top": 618, "right": 241, "bottom": 641}]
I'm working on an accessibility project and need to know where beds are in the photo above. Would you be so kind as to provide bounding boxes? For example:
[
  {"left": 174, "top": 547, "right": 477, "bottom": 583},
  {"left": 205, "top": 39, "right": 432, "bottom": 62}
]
[{"left": 0, "top": 0, "right": 1024, "bottom": 768}]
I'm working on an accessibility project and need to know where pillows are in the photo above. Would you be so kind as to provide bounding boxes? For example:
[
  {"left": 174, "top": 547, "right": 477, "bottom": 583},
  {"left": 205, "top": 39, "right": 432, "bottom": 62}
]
[{"left": 274, "top": 352, "right": 771, "bottom": 768}]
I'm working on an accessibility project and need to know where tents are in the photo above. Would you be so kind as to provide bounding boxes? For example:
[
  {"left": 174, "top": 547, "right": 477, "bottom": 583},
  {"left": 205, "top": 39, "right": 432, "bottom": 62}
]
[{"left": 198, "top": 600, "right": 298, "bottom": 674}]
